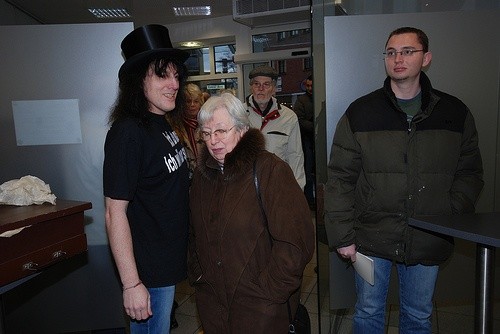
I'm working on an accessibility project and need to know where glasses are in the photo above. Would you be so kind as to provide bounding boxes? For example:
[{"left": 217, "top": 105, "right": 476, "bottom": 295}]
[
  {"left": 250, "top": 81, "right": 273, "bottom": 88},
  {"left": 383, "top": 49, "right": 425, "bottom": 57},
  {"left": 199, "top": 126, "right": 236, "bottom": 141}
]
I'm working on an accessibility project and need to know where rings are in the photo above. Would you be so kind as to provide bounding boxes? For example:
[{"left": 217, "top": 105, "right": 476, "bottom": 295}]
[{"left": 130, "top": 312, "right": 135, "bottom": 317}]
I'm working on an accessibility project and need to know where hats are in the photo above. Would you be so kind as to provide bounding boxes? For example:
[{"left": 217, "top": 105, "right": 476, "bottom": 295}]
[
  {"left": 249, "top": 65, "right": 277, "bottom": 82},
  {"left": 118, "top": 24, "right": 190, "bottom": 85}
]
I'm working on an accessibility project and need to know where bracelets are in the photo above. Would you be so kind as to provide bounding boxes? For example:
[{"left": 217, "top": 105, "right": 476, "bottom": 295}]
[{"left": 121, "top": 280, "right": 143, "bottom": 290}]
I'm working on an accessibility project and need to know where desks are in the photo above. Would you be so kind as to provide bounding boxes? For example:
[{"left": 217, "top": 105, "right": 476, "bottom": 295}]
[{"left": 410, "top": 213, "right": 500, "bottom": 333}]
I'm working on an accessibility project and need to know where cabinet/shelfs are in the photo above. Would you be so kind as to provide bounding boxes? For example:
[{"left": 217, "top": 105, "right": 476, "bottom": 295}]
[{"left": 0, "top": 197, "right": 95, "bottom": 282}]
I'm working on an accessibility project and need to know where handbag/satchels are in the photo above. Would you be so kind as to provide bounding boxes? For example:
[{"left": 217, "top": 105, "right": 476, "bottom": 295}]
[{"left": 288, "top": 304, "right": 311, "bottom": 334}]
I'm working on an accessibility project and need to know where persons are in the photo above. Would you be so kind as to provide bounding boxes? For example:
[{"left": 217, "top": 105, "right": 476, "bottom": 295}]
[
  {"left": 293, "top": 74, "right": 316, "bottom": 210},
  {"left": 242, "top": 65, "right": 307, "bottom": 194},
  {"left": 103, "top": 45, "right": 192, "bottom": 334},
  {"left": 165, "top": 83, "right": 207, "bottom": 195},
  {"left": 321, "top": 24, "right": 485, "bottom": 334},
  {"left": 200, "top": 93, "right": 211, "bottom": 104},
  {"left": 185, "top": 90, "right": 316, "bottom": 334}
]
